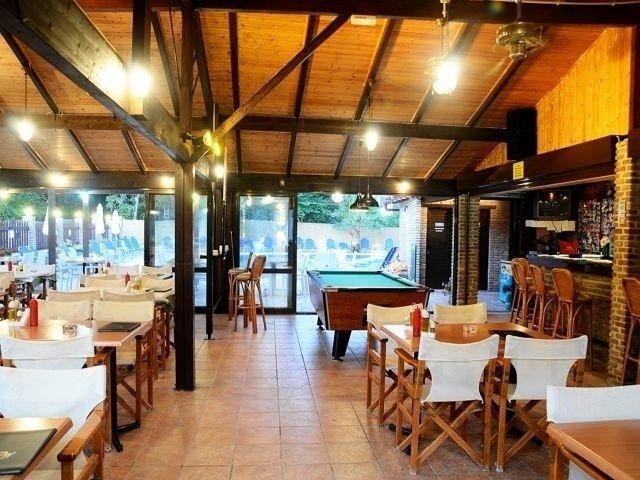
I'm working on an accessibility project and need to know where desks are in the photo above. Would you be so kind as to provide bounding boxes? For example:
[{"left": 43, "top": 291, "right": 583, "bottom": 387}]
[
  {"left": 546, "top": 420, "right": 640, "bottom": 480},
  {"left": 306, "top": 269, "right": 434, "bottom": 363},
  {"left": 382, "top": 322, "right": 558, "bottom": 456}
]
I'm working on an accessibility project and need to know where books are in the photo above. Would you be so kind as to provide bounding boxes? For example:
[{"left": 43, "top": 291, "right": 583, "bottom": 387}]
[
  {"left": 0, "top": 428, "right": 57, "bottom": 474},
  {"left": 147, "top": 287, "right": 172, "bottom": 292},
  {"left": 98, "top": 322, "right": 141, "bottom": 331},
  {"left": 489, "top": 329, "right": 533, "bottom": 340}
]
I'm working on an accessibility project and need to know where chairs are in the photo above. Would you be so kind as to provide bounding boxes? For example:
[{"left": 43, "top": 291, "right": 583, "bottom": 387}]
[
  {"left": 384, "top": 238, "right": 398, "bottom": 253},
  {"left": 283, "top": 236, "right": 372, "bottom": 297},
  {"left": 394, "top": 334, "right": 500, "bottom": 475},
  {"left": 545, "top": 384, "right": 640, "bottom": 480},
  {"left": 511, "top": 258, "right": 640, "bottom": 384},
  {"left": 228, "top": 235, "right": 273, "bottom": 334},
  {"left": 0, "top": 235, "right": 177, "bottom": 480},
  {"left": 491, "top": 335, "right": 589, "bottom": 474},
  {"left": 434, "top": 303, "right": 488, "bottom": 324},
  {"left": 367, "top": 303, "right": 423, "bottom": 427}
]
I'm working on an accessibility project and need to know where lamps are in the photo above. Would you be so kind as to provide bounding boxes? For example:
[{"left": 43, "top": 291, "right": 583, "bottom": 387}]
[
  {"left": 10, "top": 62, "right": 40, "bottom": 144},
  {"left": 359, "top": 75, "right": 381, "bottom": 152},
  {"left": 348, "top": 152, "right": 380, "bottom": 212},
  {"left": 423, "top": 16, "right": 463, "bottom": 97}
]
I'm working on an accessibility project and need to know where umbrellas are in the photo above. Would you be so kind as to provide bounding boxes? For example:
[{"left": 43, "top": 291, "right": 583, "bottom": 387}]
[
  {"left": 95, "top": 203, "right": 105, "bottom": 245},
  {"left": 112, "top": 208, "right": 120, "bottom": 248},
  {"left": 42, "top": 207, "right": 49, "bottom": 236}
]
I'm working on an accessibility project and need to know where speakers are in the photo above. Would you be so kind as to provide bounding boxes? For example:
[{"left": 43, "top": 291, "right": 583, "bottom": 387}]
[{"left": 506, "top": 106, "right": 537, "bottom": 160}]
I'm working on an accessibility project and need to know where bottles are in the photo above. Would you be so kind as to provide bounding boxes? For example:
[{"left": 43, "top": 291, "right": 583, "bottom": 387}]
[
  {"left": 406, "top": 304, "right": 430, "bottom": 337},
  {"left": 29, "top": 296, "right": 39, "bottom": 326},
  {"left": 124, "top": 271, "right": 130, "bottom": 288},
  {"left": 577, "top": 196, "right": 613, "bottom": 254},
  {"left": 83, "top": 261, "right": 111, "bottom": 276},
  {"left": 63, "top": 322, "right": 78, "bottom": 338},
  {"left": 8, "top": 300, "right": 18, "bottom": 321},
  {"left": 134, "top": 279, "right": 143, "bottom": 292},
  {"left": 600, "top": 234, "right": 610, "bottom": 258},
  {"left": 8, "top": 258, "right": 12, "bottom": 271},
  {"left": 16, "top": 259, "right": 23, "bottom": 272}
]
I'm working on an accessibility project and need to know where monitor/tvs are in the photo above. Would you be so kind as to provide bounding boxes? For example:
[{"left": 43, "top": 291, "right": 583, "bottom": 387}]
[{"left": 533, "top": 190, "right": 572, "bottom": 221}]
